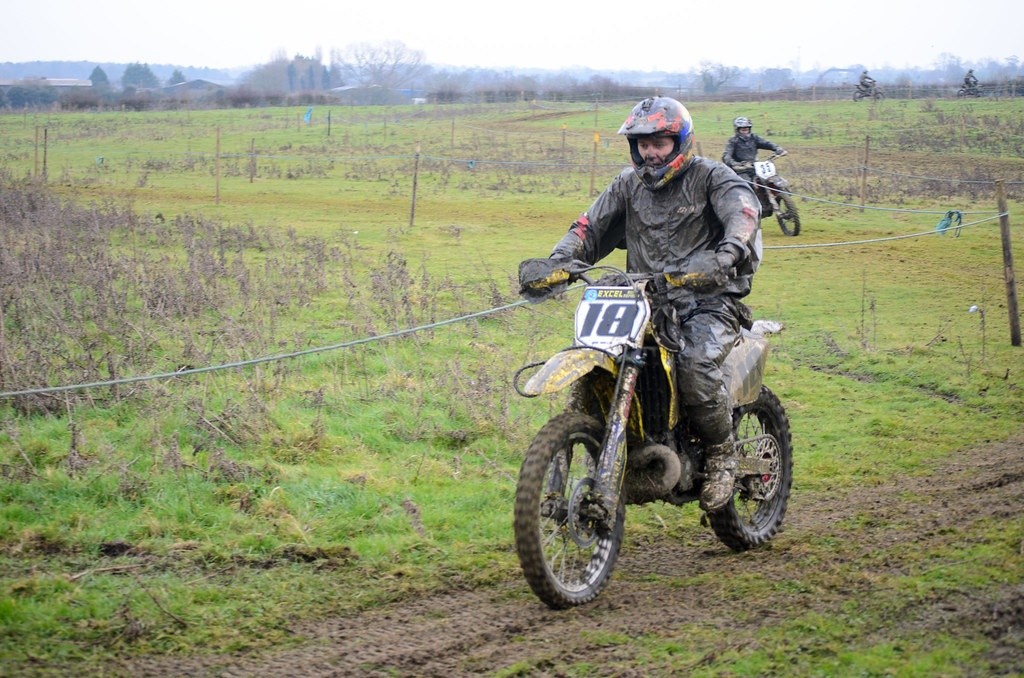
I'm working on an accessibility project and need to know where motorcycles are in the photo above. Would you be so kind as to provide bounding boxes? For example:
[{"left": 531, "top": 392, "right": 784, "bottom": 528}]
[
  {"left": 724, "top": 150, "right": 800, "bottom": 236},
  {"left": 957, "top": 80, "right": 987, "bottom": 98},
  {"left": 852, "top": 80, "right": 886, "bottom": 102},
  {"left": 513, "top": 254, "right": 795, "bottom": 609}
]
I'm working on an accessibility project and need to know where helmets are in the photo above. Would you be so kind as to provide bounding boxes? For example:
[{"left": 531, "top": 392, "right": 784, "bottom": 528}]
[
  {"left": 734, "top": 117, "right": 752, "bottom": 141},
  {"left": 618, "top": 97, "right": 695, "bottom": 191}
]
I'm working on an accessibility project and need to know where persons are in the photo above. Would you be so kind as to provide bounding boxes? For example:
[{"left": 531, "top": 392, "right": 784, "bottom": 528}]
[
  {"left": 965, "top": 69, "right": 980, "bottom": 97},
  {"left": 518, "top": 96, "right": 762, "bottom": 511},
  {"left": 721, "top": 117, "right": 783, "bottom": 186},
  {"left": 860, "top": 70, "right": 875, "bottom": 93}
]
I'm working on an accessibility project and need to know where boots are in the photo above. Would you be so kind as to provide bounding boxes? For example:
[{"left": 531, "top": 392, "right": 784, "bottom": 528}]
[{"left": 686, "top": 386, "right": 738, "bottom": 512}]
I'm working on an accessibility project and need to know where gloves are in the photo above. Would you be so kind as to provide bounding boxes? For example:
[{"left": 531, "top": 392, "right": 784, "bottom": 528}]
[
  {"left": 729, "top": 159, "right": 742, "bottom": 166},
  {"left": 714, "top": 250, "right": 736, "bottom": 274},
  {"left": 776, "top": 147, "right": 783, "bottom": 154}
]
